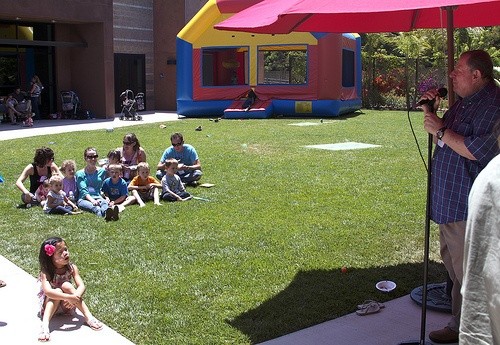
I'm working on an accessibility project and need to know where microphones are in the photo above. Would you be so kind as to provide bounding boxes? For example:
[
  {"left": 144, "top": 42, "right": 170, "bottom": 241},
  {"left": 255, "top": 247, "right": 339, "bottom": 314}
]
[{"left": 409, "top": 88, "right": 448, "bottom": 111}]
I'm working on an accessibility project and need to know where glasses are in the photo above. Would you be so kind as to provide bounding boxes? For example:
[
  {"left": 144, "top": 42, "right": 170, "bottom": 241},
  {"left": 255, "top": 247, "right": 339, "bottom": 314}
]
[
  {"left": 172, "top": 141, "right": 183, "bottom": 147},
  {"left": 86, "top": 155, "right": 99, "bottom": 159},
  {"left": 122, "top": 141, "right": 134, "bottom": 145},
  {"left": 50, "top": 158, "right": 56, "bottom": 162}
]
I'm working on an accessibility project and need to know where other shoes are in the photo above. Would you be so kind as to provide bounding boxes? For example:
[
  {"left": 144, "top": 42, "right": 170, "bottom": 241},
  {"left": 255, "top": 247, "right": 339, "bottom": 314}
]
[
  {"left": 428, "top": 326, "right": 460, "bottom": 344},
  {"left": 112, "top": 206, "right": 119, "bottom": 221},
  {"left": 104, "top": 207, "right": 114, "bottom": 222}
]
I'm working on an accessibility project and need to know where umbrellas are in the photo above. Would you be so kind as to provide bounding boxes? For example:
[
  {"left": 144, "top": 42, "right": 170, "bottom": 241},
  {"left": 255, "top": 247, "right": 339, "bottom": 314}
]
[{"left": 212, "top": 0, "right": 500, "bottom": 314}]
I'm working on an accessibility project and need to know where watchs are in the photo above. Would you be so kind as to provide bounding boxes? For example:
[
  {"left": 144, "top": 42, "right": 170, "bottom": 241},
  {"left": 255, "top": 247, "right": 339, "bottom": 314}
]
[{"left": 436, "top": 127, "right": 448, "bottom": 140}]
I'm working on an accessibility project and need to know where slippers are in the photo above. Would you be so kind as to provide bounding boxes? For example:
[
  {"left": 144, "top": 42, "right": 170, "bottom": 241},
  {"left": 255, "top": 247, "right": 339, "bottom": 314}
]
[
  {"left": 355, "top": 302, "right": 380, "bottom": 316},
  {"left": 356, "top": 300, "right": 385, "bottom": 310}
]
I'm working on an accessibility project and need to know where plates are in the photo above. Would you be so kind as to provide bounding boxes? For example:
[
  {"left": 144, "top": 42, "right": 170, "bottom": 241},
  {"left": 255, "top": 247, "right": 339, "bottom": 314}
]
[{"left": 376, "top": 280, "right": 397, "bottom": 291}]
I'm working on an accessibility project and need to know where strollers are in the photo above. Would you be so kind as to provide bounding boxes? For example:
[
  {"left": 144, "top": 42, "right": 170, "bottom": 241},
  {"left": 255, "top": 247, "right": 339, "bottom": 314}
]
[
  {"left": 119, "top": 89, "right": 144, "bottom": 121},
  {"left": 6, "top": 98, "right": 36, "bottom": 123},
  {"left": 61, "top": 91, "right": 81, "bottom": 120}
]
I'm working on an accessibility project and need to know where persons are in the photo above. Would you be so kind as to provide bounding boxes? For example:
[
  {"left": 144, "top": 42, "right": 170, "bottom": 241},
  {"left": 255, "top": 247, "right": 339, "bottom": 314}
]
[
  {"left": 5, "top": 75, "right": 42, "bottom": 127},
  {"left": 115, "top": 133, "right": 146, "bottom": 187},
  {"left": 156, "top": 132, "right": 202, "bottom": 188},
  {"left": 34, "top": 146, "right": 137, "bottom": 221},
  {"left": 417, "top": 50, "right": 500, "bottom": 345},
  {"left": 127, "top": 162, "right": 163, "bottom": 208},
  {"left": 36, "top": 236, "right": 103, "bottom": 342},
  {"left": 15, "top": 146, "right": 65, "bottom": 208},
  {"left": 160, "top": 158, "right": 193, "bottom": 202}
]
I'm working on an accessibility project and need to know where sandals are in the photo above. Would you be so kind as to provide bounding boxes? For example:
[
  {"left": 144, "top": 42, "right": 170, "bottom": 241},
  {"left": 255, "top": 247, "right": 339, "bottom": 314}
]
[
  {"left": 84, "top": 319, "right": 103, "bottom": 331},
  {"left": 37, "top": 326, "right": 51, "bottom": 343}
]
